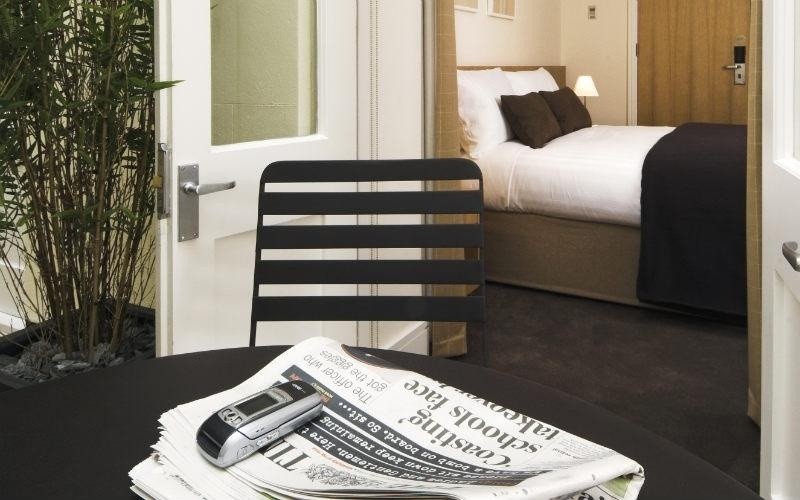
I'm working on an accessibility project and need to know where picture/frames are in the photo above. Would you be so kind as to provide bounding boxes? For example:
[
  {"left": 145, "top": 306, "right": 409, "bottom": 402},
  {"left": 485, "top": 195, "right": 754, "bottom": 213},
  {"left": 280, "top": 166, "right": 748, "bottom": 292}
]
[{"left": 453, "top": 0, "right": 517, "bottom": 21}]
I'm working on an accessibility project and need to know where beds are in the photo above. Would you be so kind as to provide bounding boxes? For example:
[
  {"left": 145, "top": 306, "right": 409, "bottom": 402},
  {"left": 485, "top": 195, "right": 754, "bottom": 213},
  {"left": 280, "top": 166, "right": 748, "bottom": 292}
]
[{"left": 454, "top": 63, "right": 747, "bottom": 325}]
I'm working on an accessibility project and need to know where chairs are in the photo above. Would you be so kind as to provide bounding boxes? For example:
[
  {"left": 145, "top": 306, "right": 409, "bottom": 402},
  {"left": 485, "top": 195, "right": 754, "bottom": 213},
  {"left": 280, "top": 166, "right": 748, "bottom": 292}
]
[{"left": 246, "top": 158, "right": 489, "bottom": 366}]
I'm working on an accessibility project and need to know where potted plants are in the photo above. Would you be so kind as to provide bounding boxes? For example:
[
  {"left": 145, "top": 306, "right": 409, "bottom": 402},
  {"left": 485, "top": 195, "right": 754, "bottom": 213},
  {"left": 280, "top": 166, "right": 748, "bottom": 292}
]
[{"left": 0, "top": 2, "right": 183, "bottom": 380}]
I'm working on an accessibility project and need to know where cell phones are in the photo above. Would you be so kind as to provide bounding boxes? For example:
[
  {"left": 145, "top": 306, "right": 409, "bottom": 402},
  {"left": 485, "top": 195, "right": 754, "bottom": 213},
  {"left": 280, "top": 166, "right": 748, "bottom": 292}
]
[{"left": 196, "top": 379, "right": 324, "bottom": 468}]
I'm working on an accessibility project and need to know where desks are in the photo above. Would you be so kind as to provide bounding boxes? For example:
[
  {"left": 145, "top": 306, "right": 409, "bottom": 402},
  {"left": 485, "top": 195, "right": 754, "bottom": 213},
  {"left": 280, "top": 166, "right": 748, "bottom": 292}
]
[{"left": 1, "top": 342, "right": 762, "bottom": 500}]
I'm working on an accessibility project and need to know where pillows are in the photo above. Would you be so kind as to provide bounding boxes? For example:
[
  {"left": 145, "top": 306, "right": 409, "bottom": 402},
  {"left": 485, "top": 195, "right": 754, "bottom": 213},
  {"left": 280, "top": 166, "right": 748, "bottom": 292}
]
[{"left": 455, "top": 66, "right": 594, "bottom": 160}]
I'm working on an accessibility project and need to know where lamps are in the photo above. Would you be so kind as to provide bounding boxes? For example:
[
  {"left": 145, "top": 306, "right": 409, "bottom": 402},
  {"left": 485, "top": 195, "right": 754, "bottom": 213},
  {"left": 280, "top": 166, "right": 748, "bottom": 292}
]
[{"left": 571, "top": 74, "right": 600, "bottom": 112}]
